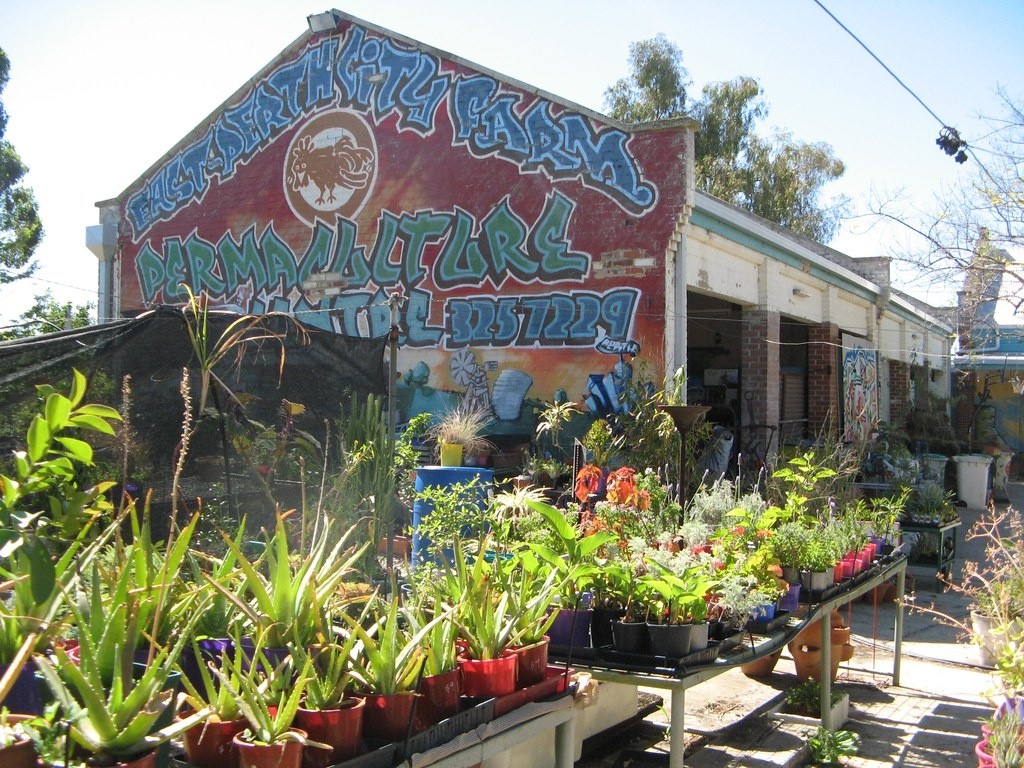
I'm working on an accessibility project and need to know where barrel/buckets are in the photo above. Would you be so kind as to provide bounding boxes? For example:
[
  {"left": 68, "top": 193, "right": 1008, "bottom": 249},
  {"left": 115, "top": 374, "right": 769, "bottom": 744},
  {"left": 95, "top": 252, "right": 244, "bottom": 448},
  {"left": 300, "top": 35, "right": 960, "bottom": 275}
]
[{"left": 411, "top": 465, "right": 494, "bottom": 574}]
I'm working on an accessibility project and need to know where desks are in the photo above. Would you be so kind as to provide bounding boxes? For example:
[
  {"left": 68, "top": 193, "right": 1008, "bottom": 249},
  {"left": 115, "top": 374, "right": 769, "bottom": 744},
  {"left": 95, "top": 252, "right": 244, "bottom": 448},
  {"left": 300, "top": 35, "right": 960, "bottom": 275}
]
[
  {"left": 390, "top": 690, "right": 576, "bottom": 768},
  {"left": 555, "top": 557, "right": 908, "bottom": 768}
]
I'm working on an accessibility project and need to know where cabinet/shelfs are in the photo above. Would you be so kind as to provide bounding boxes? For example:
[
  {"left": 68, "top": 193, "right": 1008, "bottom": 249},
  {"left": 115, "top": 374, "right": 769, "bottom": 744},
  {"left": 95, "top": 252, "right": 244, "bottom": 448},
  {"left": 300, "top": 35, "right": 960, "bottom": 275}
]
[{"left": 901, "top": 522, "right": 962, "bottom": 593}]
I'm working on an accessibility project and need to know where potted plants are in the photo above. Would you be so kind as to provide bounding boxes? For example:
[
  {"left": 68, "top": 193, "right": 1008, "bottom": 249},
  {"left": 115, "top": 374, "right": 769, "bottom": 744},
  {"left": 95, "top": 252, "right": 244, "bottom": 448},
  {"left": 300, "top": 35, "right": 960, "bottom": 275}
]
[
  {"left": 434, "top": 398, "right": 484, "bottom": 468},
  {"left": 911, "top": 486, "right": 955, "bottom": 564},
  {"left": 0, "top": 509, "right": 561, "bottom": 768},
  {"left": 981, "top": 715, "right": 1024, "bottom": 742},
  {"left": 975, "top": 726, "right": 1024, "bottom": 768},
  {"left": 764, "top": 676, "right": 850, "bottom": 729},
  {"left": 968, "top": 581, "right": 1018, "bottom": 668}
]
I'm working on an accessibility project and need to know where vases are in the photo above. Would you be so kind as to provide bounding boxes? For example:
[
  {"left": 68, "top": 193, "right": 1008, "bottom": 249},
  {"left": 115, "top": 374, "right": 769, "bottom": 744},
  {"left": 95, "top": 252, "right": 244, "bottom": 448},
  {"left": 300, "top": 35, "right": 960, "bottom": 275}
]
[{"left": 547, "top": 536, "right": 892, "bottom": 662}]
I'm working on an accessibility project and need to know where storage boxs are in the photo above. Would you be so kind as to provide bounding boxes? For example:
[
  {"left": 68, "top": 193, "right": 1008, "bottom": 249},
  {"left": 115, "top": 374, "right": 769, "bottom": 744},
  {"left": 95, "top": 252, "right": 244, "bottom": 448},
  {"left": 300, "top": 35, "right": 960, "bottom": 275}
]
[{"left": 580, "top": 681, "right": 639, "bottom": 742}]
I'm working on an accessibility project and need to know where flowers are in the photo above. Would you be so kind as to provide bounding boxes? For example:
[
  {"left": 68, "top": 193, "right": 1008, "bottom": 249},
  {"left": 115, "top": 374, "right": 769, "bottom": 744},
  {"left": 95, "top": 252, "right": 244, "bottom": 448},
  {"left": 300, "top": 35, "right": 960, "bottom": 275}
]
[{"left": 536, "top": 447, "right": 913, "bottom": 625}]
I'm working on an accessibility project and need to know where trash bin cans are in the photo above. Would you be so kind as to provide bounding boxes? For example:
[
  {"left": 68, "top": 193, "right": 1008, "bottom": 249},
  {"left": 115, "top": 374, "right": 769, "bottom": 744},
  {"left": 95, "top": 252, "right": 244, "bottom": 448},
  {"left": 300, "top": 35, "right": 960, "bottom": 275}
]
[
  {"left": 918, "top": 452, "right": 949, "bottom": 493},
  {"left": 952, "top": 453, "right": 994, "bottom": 510},
  {"left": 993, "top": 450, "right": 1016, "bottom": 489}
]
